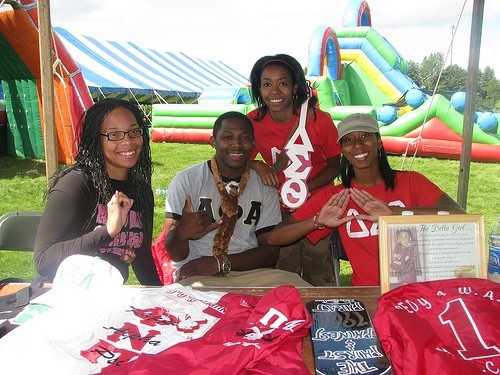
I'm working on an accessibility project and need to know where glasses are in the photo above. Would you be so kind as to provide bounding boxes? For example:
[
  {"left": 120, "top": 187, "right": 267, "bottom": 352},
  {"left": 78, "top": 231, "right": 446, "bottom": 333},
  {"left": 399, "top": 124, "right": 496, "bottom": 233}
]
[{"left": 99, "top": 127, "right": 144, "bottom": 141}]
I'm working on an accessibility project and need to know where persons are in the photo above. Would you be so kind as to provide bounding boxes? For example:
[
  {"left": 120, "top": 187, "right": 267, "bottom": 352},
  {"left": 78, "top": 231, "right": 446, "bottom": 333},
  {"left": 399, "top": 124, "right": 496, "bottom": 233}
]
[
  {"left": 390, "top": 231, "right": 416, "bottom": 285},
  {"left": 267, "top": 113, "right": 468, "bottom": 286},
  {"left": 32, "top": 98, "right": 163, "bottom": 286},
  {"left": 243, "top": 54, "right": 343, "bottom": 287},
  {"left": 164, "top": 111, "right": 282, "bottom": 282}
]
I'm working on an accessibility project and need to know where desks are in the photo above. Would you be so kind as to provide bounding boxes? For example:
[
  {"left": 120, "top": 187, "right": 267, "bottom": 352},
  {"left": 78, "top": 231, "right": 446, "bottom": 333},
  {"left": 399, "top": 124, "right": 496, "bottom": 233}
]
[{"left": 0, "top": 284, "right": 394, "bottom": 375}]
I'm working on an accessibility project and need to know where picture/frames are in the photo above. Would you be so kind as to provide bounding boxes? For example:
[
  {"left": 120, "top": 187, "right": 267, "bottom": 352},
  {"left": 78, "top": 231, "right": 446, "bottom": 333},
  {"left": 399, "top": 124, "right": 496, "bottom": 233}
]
[{"left": 379, "top": 214, "right": 487, "bottom": 295}]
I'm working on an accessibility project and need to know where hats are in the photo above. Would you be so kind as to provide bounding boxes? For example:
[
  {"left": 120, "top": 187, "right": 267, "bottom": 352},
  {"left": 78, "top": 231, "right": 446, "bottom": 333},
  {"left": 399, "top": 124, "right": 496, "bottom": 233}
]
[{"left": 336, "top": 113, "right": 379, "bottom": 144}]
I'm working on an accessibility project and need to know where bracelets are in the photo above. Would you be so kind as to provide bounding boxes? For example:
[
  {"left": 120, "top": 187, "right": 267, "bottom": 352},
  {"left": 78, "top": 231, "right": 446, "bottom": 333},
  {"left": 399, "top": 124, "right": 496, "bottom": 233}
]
[
  {"left": 212, "top": 254, "right": 221, "bottom": 277},
  {"left": 254, "top": 160, "right": 263, "bottom": 169},
  {"left": 313, "top": 212, "right": 326, "bottom": 230}
]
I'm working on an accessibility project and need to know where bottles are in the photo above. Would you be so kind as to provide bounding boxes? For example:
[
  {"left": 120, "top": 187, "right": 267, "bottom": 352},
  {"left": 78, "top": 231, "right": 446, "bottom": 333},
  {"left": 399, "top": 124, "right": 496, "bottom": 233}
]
[{"left": 488, "top": 219, "right": 500, "bottom": 284}]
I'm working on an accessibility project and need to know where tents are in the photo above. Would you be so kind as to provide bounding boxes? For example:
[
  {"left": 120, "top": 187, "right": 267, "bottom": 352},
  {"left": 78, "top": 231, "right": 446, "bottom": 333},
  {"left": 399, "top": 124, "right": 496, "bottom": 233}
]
[{"left": 52, "top": 25, "right": 252, "bottom": 127}]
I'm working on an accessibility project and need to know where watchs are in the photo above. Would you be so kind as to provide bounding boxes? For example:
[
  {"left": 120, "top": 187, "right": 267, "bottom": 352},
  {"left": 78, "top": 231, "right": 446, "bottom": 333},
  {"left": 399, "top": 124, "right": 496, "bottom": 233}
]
[{"left": 219, "top": 254, "right": 232, "bottom": 277}]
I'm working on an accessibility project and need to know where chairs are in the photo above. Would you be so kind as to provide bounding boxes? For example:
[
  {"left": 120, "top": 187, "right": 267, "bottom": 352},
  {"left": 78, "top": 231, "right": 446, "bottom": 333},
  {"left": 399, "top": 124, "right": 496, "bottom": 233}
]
[
  {"left": 330, "top": 230, "right": 349, "bottom": 287},
  {"left": 0, "top": 211, "right": 42, "bottom": 282}
]
